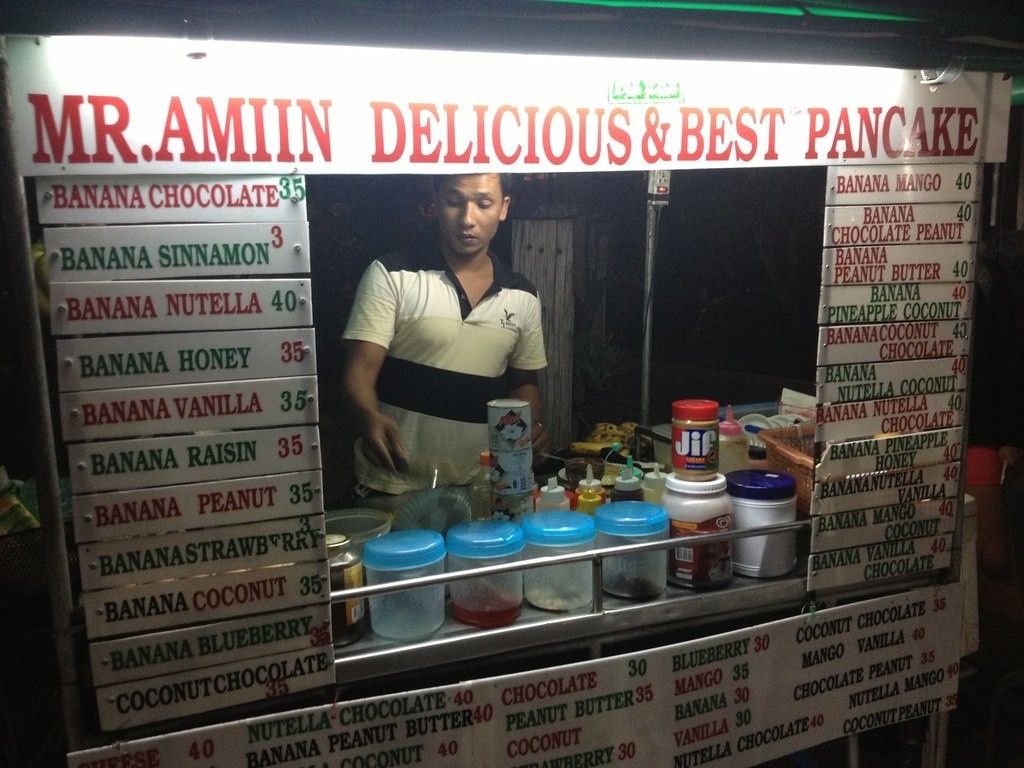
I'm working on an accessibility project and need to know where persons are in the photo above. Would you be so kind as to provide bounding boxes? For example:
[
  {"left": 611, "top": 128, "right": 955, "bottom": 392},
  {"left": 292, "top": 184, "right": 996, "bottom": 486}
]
[
  {"left": 693, "top": 220, "right": 1024, "bottom": 681},
  {"left": 342, "top": 173, "right": 557, "bottom": 508}
]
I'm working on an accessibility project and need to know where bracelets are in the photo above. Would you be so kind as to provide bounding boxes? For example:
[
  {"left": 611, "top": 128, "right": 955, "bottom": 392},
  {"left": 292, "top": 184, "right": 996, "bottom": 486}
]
[{"left": 532, "top": 419, "right": 544, "bottom": 429}]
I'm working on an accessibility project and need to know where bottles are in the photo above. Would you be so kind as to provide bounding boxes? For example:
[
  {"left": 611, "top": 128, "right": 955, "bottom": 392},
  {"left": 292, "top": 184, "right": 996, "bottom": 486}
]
[
  {"left": 719, "top": 405, "right": 751, "bottom": 476},
  {"left": 575, "top": 464, "right": 606, "bottom": 510},
  {"left": 611, "top": 470, "right": 644, "bottom": 503},
  {"left": 326, "top": 534, "right": 363, "bottom": 648},
  {"left": 536, "top": 476, "right": 570, "bottom": 512},
  {"left": 620, "top": 455, "right": 644, "bottom": 480},
  {"left": 471, "top": 452, "right": 491, "bottom": 516},
  {"left": 576, "top": 486, "right": 603, "bottom": 514},
  {"left": 643, "top": 462, "right": 668, "bottom": 505},
  {"left": 661, "top": 472, "right": 732, "bottom": 589},
  {"left": 532, "top": 473, "right": 540, "bottom": 508}
]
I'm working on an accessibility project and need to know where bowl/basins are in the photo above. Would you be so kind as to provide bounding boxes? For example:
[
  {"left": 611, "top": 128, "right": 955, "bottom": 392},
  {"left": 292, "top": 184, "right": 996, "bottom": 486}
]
[{"left": 392, "top": 487, "right": 471, "bottom": 535}]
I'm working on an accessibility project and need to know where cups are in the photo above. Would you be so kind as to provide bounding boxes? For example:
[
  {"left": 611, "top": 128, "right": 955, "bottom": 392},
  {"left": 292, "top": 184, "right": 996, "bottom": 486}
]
[
  {"left": 521, "top": 511, "right": 597, "bottom": 611},
  {"left": 325, "top": 508, "right": 392, "bottom": 558},
  {"left": 725, "top": 469, "right": 796, "bottom": 577},
  {"left": 447, "top": 519, "right": 525, "bottom": 625},
  {"left": 365, "top": 530, "right": 447, "bottom": 641},
  {"left": 595, "top": 500, "right": 670, "bottom": 599}
]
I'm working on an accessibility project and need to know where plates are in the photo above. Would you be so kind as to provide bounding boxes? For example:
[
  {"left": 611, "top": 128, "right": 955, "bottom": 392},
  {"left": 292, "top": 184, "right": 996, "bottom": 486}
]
[{"left": 737, "top": 413, "right": 806, "bottom": 448}]
